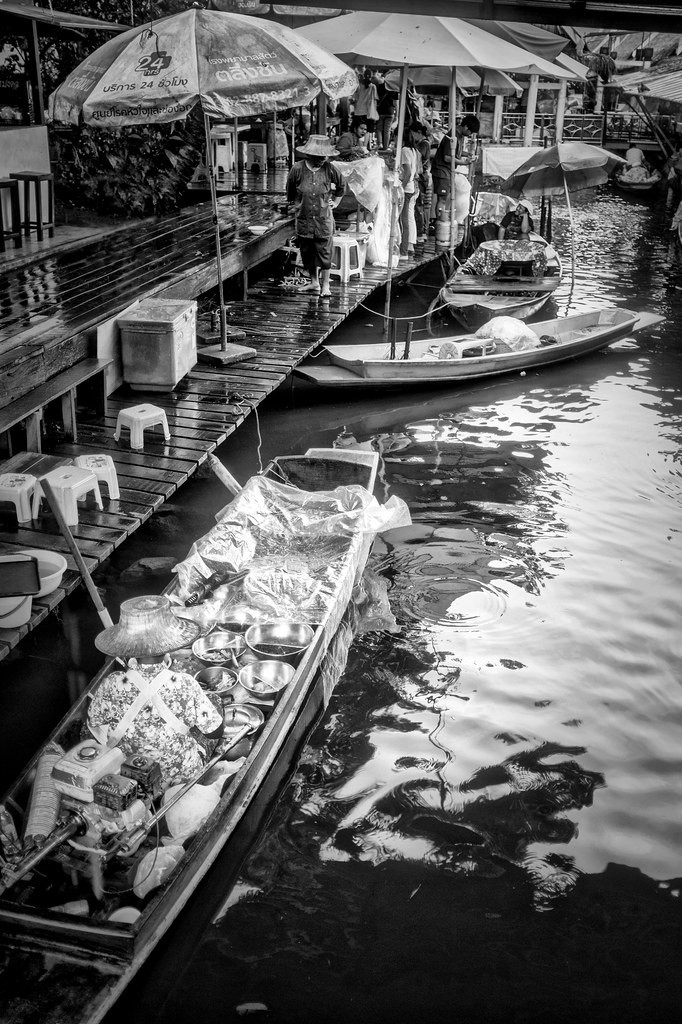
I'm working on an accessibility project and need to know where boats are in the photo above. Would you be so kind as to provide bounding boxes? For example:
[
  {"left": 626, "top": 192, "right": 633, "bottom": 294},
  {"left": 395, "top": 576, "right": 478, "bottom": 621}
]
[
  {"left": 288, "top": 306, "right": 667, "bottom": 386},
  {"left": 614, "top": 159, "right": 663, "bottom": 191},
  {"left": 0, "top": 445, "right": 383, "bottom": 1024},
  {"left": 439, "top": 229, "right": 564, "bottom": 335}
]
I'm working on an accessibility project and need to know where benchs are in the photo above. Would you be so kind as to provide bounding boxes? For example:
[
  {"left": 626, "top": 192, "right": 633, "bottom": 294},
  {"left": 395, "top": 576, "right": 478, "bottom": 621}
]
[{"left": 0, "top": 357, "right": 114, "bottom": 454}]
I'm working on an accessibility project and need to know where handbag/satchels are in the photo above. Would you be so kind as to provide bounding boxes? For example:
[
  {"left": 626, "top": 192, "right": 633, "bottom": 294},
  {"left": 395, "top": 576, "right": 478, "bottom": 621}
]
[{"left": 366, "top": 84, "right": 379, "bottom": 121}]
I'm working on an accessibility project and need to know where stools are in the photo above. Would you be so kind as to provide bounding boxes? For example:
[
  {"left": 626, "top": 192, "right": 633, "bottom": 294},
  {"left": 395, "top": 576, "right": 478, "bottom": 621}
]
[
  {"left": 0, "top": 473, "right": 43, "bottom": 524},
  {"left": 31, "top": 465, "right": 103, "bottom": 526},
  {"left": 248, "top": 143, "right": 267, "bottom": 170},
  {"left": 439, "top": 338, "right": 493, "bottom": 359},
  {"left": 113, "top": 403, "right": 171, "bottom": 449},
  {"left": 0, "top": 171, "right": 55, "bottom": 252},
  {"left": 74, "top": 454, "right": 120, "bottom": 502},
  {"left": 319, "top": 237, "right": 364, "bottom": 283}
]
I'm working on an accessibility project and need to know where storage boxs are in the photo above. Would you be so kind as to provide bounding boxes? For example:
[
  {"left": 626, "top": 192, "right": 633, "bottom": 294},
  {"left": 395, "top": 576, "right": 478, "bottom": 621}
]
[{"left": 117, "top": 297, "right": 197, "bottom": 393}]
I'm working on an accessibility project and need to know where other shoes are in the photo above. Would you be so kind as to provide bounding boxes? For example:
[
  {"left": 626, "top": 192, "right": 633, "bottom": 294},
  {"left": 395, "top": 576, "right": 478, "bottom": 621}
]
[
  {"left": 399, "top": 247, "right": 408, "bottom": 260},
  {"left": 408, "top": 250, "right": 414, "bottom": 261},
  {"left": 417, "top": 237, "right": 425, "bottom": 245},
  {"left": 422, "top": 234, "right": 428, "bottom": 242},
  {"left": 378, "top": 143, "right": 384, "bottom": 150}
]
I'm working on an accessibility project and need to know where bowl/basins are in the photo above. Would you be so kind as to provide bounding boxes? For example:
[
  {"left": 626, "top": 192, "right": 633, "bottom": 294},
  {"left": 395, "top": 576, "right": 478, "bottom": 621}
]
[
  {"left": 248, "top": 225, "right": 268, "bottom": 235},
  {"left": 17, "top": 549, "right": 68, "bottom": 598},
  {"left": 192, "top": 603, "right": 315, "bottom": 701}
]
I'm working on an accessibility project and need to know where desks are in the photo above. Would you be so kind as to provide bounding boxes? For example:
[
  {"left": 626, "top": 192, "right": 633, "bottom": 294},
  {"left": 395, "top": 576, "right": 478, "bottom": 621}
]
[{"left": 210, "top": 123, "right": 250, "bottom": 175}]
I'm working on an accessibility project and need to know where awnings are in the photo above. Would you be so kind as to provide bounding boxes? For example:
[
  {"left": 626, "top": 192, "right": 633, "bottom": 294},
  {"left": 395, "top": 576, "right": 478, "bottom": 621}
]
[
  {"left": 612, "top": 31, "right": 651, "bottom": 60},
  {"left": 642, "top": 32, "right": 680, "bottom": 61},
  {"left": 577, "top": 28, "right": 610, "bottom": 55},
  {"left": 597, "top": 58, "right": 682, "bottom": 162}
]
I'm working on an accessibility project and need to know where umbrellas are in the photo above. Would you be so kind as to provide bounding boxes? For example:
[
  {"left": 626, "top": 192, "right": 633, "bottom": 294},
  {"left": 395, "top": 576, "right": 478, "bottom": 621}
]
[
  {"left": 294, "top": 13, "right": 590, "bottom": 333},
  {"left": 383, "top": 64, "right": 524, "bottom": 134},
  {"left": 48, "top": 6, "right": 360, "bottom": 350},
  {"left": 499, "top": 141, "right": 629, "bottom": 236}
]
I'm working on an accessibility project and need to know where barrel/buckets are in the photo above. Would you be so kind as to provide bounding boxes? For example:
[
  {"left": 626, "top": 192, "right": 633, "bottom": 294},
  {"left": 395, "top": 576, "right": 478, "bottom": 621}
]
[{"left": 0, "top": 553, "right": 33, "bottom": 629}]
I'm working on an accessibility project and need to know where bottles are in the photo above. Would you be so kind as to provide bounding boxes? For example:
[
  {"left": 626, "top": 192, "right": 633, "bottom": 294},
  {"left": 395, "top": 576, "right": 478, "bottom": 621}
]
[{"left": 0, "top": 804, "right": 24, "bottom": 864}]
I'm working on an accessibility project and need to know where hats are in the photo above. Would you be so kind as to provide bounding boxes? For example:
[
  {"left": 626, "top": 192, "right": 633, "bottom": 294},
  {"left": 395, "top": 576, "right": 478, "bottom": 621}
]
[
  {"left": 95, "top": 594, "right": 203, "bottom": 659},
  {"left": 295, "top": 134, "right": 340, "bottom": 157},
  {"left": 519, "top": 199, "right": 534, "bottom": 216}
]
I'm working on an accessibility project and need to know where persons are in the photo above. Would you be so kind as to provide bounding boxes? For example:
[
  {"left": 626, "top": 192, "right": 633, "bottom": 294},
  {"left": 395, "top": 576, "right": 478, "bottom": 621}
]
[
  {"left": 335, "top": 120, "right": 368, "bottom": 160},
  {"left": 625, "top": 142, "right": 647, "bottom": 171},
  {"left": 87, "top": 594, "right": 224, "bottom": 792},
  {"left": 425, "top": 95, "right": 435, "bottom": 109},
  {"left": 498, "top": 199, "right": 535, "bottom": 240},
  {"left": 400, "top": 130, "right": 417, "bottom": 261},
  {"left": 407, "top": 147, "right": 423, "bottom": 252},
  {"left": 286, "top": 134, "right": 347, "bottom": 296},
  {"left": 553, "top": 86, "right": 572, "bottom": 116},
  {"left": 371, "top": 76, "right": 395, "bottom": 150},
  {"left": 421, "top": 101, "right": 441, "bottom": 133},
  {"left": 389, "top": 140, "right": 395, "bottom": 168},
  {"left": 353, "top": 69, "right": 379, "bottom": 149},
  {"left": 411, "top": 120, "right": 430, "bottom": 242},
  {"left": 430, "top": 114, "right": 480, "bottom": 219},
  {"left": 514, "top": 101, "right": 523, "bottom": 113}
]
